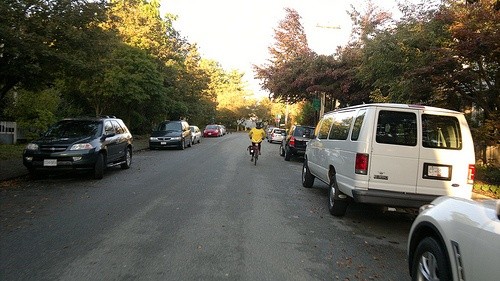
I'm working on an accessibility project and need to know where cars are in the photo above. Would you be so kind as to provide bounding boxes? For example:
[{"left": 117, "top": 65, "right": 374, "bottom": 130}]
[
  {"left": 204, "top": 124, "right": 220, "bottom": 137},
  {"left": 280, "top": 124, "right": 317, "bottom": 162},
  {"left": 218, "top": 125, "right": 227, "bottom": 136},
  {"left": 406, "top": 195, "right": 500, "bottom": 281},
  {"left": 268, "top": 128, "right": 286, "bottom": 144},
  {"left": 263, "top": 125, "right": 276, "bottom": 140},
  {"left": 189, "top": 125, "right": 202, "bottom": 145}
]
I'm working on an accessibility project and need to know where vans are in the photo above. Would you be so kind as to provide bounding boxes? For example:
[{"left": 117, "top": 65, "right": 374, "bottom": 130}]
[{"left": 301, "top": 100, "right": 476, "bottom": 218}]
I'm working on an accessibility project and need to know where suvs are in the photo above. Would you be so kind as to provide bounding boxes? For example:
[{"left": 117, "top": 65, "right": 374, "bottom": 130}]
[
  {"left": 22, "top": 113, "right": 134, "bottom": 180},
  {"left": 149, "top": 119, "right": 193, "bottom": 150}
]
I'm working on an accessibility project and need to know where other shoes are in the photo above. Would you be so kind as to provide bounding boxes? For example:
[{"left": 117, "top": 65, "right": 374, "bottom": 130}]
[
  {"left": 251, "top": 159, "right": 253, "bottom": 161},
  {"left": 259, "top": 154, "right": 261, "bottom": 155}
]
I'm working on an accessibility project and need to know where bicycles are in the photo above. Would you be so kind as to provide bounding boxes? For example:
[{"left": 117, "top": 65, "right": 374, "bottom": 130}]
[{"left": 249, "top": 137, "right": 266, "bottom": 165}]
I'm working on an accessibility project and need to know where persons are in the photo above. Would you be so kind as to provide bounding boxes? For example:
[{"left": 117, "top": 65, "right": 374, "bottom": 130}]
[{"left": 249, "top": 122, "right": 265, "bottom": 161}]
